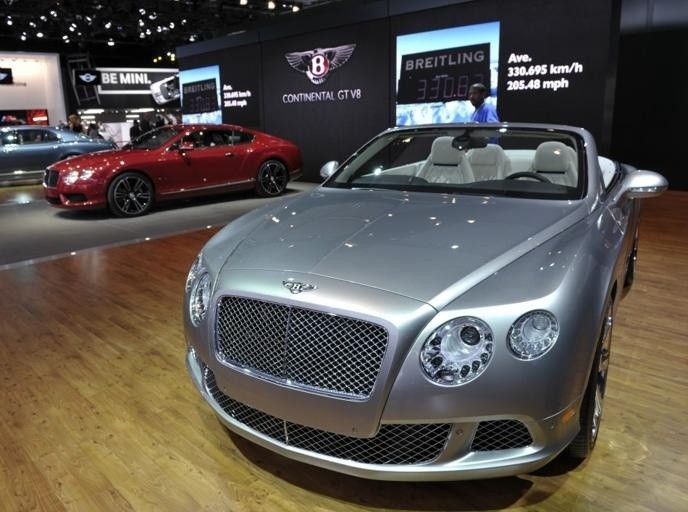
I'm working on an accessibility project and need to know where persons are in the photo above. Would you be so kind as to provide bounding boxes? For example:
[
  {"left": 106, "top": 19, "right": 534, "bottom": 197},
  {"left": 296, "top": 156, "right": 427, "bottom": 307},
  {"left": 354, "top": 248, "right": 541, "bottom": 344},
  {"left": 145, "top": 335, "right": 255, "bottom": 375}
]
[
  {"left": 60, "top": 109, "right": 183, "bottom": 144},
  {"left": 469, "top": 83, "right": 501, "bottom": 123}
]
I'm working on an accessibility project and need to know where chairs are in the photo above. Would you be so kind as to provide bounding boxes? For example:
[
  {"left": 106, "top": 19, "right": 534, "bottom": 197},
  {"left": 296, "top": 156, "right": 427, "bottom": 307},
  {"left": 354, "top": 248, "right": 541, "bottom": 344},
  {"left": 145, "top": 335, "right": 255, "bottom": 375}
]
[{"left": 414, "top": 137, "right": 577, "bottom": 184}]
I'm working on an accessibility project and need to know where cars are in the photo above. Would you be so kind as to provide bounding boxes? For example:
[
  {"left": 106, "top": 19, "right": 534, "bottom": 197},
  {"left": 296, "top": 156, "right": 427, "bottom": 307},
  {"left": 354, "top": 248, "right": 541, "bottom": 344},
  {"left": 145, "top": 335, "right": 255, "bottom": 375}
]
[
  {"left": 179, "top": 119, "right": 670, "bottom": 482},
  {"left": 42, "top": 124, "right": 306, "bottom": 219},
  {"left": 0, "top": 124, "right": 120, "bottom": 181}
]
